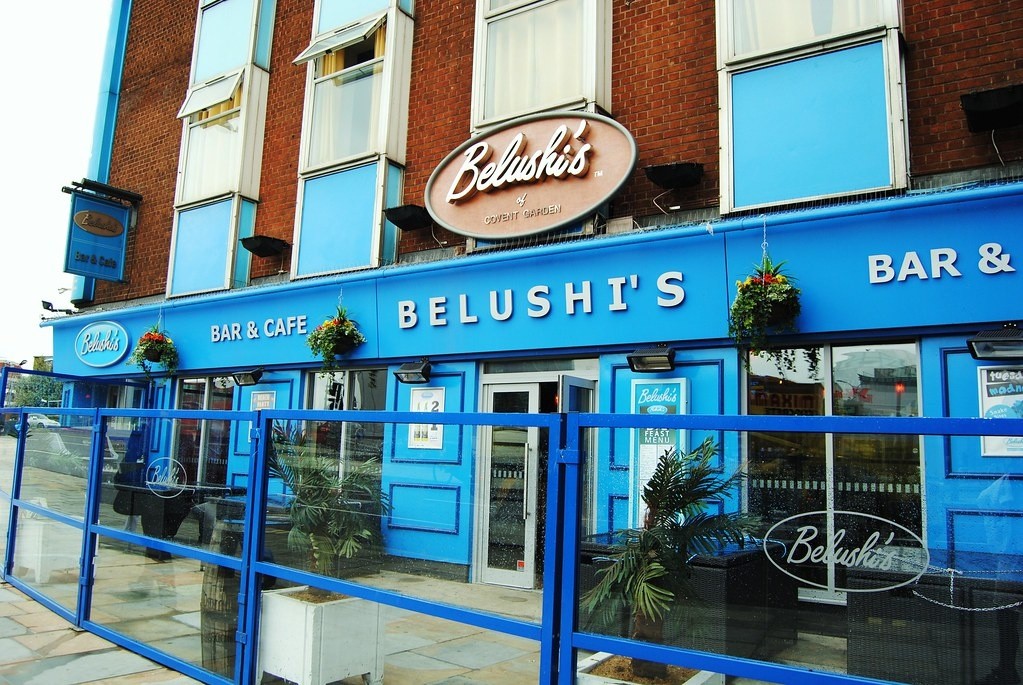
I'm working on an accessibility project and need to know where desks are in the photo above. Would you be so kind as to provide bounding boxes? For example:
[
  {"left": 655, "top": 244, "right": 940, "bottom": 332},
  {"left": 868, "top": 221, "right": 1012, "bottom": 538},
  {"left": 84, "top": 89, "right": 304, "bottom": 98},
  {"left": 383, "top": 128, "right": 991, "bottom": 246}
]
[
  {"left": 576, "top": 531, "right": 798, "bottom": 681},
  {"left": 846, "top": 545, "right": 1023, "bottom": 685},
  {"left": 99, "top": 480, "right": 382, "bottom": 580}
]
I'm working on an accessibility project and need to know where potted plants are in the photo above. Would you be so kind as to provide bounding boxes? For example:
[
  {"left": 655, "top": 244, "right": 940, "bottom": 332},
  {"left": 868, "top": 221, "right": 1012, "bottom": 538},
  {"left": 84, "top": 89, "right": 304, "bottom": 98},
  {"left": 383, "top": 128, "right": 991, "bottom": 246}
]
[
  {"left": 256, "top": 421, "right": 396, "bottom": 685},
  {"left": 575, "top": 435, "right": 765, "bottom": 685},
  {"left": 13, "top": 423, "right": 100, "bottom": 585}
]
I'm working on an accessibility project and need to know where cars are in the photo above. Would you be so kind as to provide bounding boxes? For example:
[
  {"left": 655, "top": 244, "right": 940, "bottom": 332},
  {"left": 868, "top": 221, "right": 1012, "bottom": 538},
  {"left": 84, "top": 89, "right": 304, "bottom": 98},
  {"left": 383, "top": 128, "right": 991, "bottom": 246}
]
[{"left": 4, "top": 414, "right": 61, "bottom": 439}]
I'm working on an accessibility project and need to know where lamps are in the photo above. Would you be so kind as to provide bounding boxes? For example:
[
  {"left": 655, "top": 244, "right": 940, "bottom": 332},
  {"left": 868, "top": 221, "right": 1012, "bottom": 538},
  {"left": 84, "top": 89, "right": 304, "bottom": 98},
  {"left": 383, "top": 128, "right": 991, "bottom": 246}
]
[
  {"left": 392, "top": 357, "right": 431, "bottom": 384},
  {"left": 626, "top": 343, "right": 675, "bottom": 373},
  {"left": 237, "top": 235, "right": 292, "bottom": 259},
  {"left": 640, "top": 162, "right": 705, "bottom": 189},
  {"left": 961, "top": 82, "right": 1023, "bottom": 134},
  {"left": 232, "top": 367, "right": 264, "bottom": 386},
  {"left": 42, "top": 301, "right": 83, "bottom": 315},
  {"left": 382, "top": 204, "right": 434, "bottom": 232},
  {"left": 966, "top": 322, "right": 1023, "bottom": 361}
]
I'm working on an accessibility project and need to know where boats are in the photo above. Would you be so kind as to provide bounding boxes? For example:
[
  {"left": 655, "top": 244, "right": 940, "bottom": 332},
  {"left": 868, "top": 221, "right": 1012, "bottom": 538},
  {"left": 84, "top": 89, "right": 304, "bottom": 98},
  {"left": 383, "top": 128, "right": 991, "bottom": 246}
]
[{"left": 857, "top": 365, "right": 917, "bottom": 386}]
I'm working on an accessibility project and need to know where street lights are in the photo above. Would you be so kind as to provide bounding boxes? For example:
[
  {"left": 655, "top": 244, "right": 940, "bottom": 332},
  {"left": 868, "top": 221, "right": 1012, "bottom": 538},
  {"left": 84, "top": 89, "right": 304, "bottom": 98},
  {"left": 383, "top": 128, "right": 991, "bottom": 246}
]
[{"left": 7, "top": 359, "right": 28, "bottom": 405}]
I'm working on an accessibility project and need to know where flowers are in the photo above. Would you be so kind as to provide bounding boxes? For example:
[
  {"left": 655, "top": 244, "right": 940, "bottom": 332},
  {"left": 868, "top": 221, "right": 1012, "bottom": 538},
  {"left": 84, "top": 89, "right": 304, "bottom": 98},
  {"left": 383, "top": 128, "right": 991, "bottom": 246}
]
[
  {"left": 305, "top": 306, "right": 367, "bottom": 378},
  {"left": 126, "top": 326, "right": 180, "bottom": 386},
  {"left": 728, "top": 256, "right": 801, "bottom": 375}
]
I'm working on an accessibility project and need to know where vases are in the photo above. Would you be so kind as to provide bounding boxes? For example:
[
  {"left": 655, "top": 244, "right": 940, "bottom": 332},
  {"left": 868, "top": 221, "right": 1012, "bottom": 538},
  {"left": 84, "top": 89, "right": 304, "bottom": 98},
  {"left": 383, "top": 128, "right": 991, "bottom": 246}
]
[
  {"left": 767, "top": 300, "right": 792, "bottom": 324},
  {"left": 333, "top": 341, "right": 355, "bottom": 355},
  {"left": 144, "top": 349, "right": 163, "bottom": 362}
]
